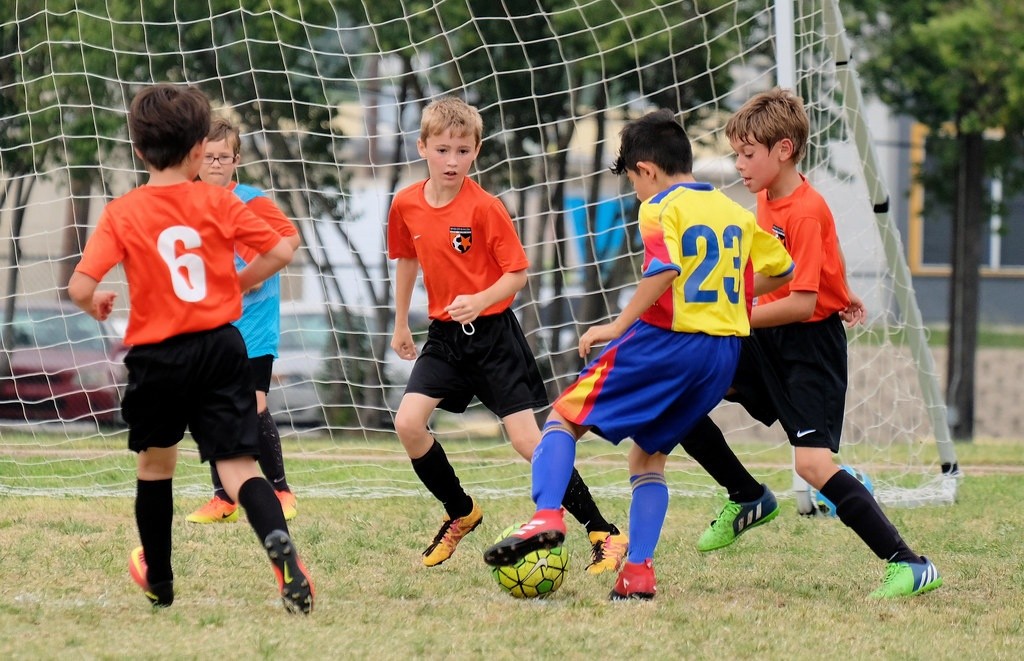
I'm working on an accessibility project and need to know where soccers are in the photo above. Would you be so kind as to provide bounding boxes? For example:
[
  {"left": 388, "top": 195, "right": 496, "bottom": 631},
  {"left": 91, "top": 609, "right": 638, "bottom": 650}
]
[
  {"left": 816, "top": 466, "right": 874, "bottom": 518},
  {"left": 490, "top": 522, "right": 569, "bottom": 600}
]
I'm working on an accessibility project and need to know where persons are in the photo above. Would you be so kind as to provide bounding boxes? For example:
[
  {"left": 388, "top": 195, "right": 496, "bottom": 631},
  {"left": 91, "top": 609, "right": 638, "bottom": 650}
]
[
  {"left": 69, "top": 83, "right": 315, "bottom": 617},
  {"left": 185, "top": 119, "right": 301, "bottom": 525},
  {"left": 484, "top": 109, "right": 794, "bottom": 600},
  {"left": 677, "top": 91, "right": 944, "bottom": 600},
  {"left": 388, "top": 97, "right": 631, "bottom": 577}
]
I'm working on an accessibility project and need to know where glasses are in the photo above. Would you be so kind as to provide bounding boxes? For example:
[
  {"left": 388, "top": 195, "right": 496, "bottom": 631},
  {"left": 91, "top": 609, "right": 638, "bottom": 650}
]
[{"left": 201, "top": 154, "right": 236, "bottom": 166}]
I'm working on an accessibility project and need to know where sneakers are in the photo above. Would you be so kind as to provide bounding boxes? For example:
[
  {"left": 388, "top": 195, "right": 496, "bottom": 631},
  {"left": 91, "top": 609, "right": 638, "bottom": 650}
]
[
  {"left": 264, "top": 529, "right": 316, "bottom": 617},
  {"left": 866, "top": 556, "right": 943, "bottom": 603},
  {"left": 275, "top": 490, "right": 297, "bottom": 520},
  {"left": 422, "top": 494, "right": 482, "bottom": 567},
  {"left": 129, "top": 546, "right": 175, "bottom": 607},
  {"left": 484, "top": 507, "right": 566, "bottom": 565},
  {"left": 585, "top": 524, "right": 629, "bottom": 575},
  {"left": 607, "top": 557, "right": 656, "bottom": 599},
  {"left": 697, "top": 482, "right": 780, "bottom": 551},
  {"left": 184, "top": 495, "right": 239, "bottom": 523}
]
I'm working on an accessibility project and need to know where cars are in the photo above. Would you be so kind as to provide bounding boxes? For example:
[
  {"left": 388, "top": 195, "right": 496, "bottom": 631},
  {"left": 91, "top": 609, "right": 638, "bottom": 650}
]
[
  {"left": 267, "top": 295, "right": 446, "bottom": 434},
  {"left": 1, "top": 295, "right": 131, "bottom": 425}
]
